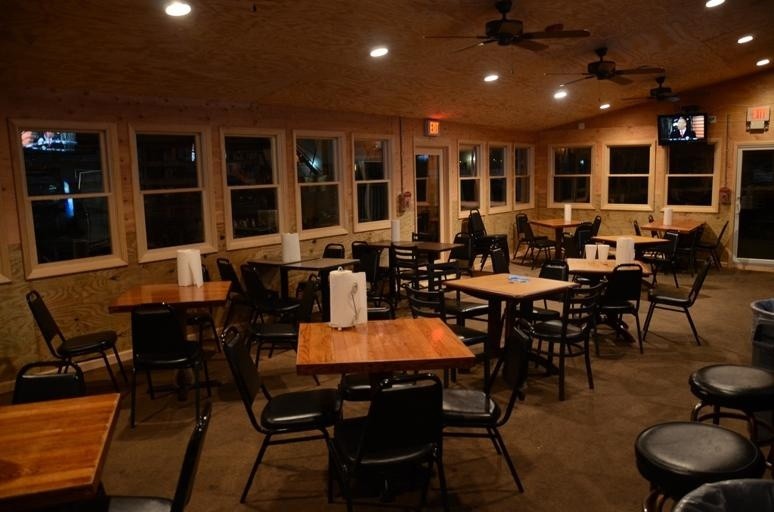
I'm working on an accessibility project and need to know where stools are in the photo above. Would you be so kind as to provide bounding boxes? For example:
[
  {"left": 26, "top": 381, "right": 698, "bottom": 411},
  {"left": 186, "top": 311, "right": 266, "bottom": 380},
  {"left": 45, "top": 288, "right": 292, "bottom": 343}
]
[{"left": 635, "top": 364, "right": 774, "bottom": 512}]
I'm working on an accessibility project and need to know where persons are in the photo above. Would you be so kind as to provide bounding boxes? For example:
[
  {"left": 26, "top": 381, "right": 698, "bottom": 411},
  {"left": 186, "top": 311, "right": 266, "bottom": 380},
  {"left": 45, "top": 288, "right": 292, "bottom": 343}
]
[
  {"left": 670, "top": 116, "right": 696, "bottom": 138},
  {"left": 33, "top": 132, "right": 64, "bottom": 149}
]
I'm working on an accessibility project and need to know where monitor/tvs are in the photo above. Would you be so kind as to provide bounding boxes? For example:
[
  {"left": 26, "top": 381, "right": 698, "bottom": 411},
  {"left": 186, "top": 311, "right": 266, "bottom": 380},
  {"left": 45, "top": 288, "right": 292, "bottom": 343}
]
[
  {"left": 657, "top": 113, "right": 707, "bottom": 147},
  {"left": 21, "top": 131, "right": 77, "bottom": 157}
]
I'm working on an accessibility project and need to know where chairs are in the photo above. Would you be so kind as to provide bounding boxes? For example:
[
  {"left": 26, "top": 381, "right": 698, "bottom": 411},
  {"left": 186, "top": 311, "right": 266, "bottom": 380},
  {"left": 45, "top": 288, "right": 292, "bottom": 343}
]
[
  {"left": 25, "top": 289, "right": 127, "bottom": 397},
  {"left": 127, "top": 302, "right": 214, "bottom": 424},
  {"left": 83, "top": 396, "right": 211, "bottom": 512},
  {"left": 296, "top": 315, "right": 476, "bottom": 479},
  {"left": 220, "top": 326, "right": 345, "bottom": 505},
  {"left": 415, "top": 316, "right": 534, "bottom": 502},
  {"left": 14, "top": 360, "right": 86, "bottom": 404},
  {"left": 127, "top": 310, "right": 211, "bottom": 434},
  {"left": 326, "top": 373, "right": 447, "bottom": 511}
]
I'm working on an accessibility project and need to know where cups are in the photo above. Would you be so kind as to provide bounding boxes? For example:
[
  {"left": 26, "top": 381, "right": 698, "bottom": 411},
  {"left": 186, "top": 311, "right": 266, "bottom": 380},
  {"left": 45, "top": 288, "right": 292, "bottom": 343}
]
[
  {"left": 585, "top": 244, "right": 597, "bottom": 262},
  {"left": 598, "top": 245, "right": 609, "bottom": 264}
]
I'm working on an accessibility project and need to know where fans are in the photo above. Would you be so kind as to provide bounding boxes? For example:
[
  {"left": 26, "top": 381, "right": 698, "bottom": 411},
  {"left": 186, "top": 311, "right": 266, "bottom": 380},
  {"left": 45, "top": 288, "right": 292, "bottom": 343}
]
[
  {"left": 547, "top": 46, "right": 665, "bottom": 87},
  {"left": 422, "top": 1, "right": 588, "bottom": 54},
  {"left": 618, "top": 78, "right": 707, "bottom": 106}
]
